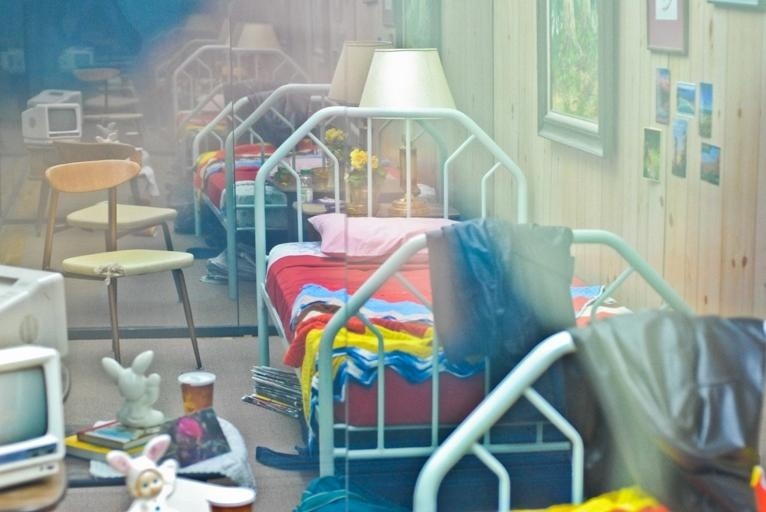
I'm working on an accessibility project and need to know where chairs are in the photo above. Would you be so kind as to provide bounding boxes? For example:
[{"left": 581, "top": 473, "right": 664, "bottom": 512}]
[
  {"left": 86, "top": 68, "right": 135, "bottom": 112},
  {"left": 75, "top": 63, "right": 147, "bottom": 145},
  {"left": 40, "top": 141, "right": 193, "bottom": 307},
  {"left": 28, "top": 160, "right": 209, "bottom": 381}
]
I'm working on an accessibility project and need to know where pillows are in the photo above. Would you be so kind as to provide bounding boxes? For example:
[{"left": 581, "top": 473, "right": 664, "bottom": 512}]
[{"left": 307, "top": 212, "right": 470, "bottom": 263}]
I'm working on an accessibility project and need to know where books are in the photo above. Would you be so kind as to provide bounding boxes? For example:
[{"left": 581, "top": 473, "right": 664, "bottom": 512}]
[
  {"left": 64, "top": 432, "right": 145, "bottom": 463},
  {"left": 241, "top": 365, "right": 302, "bottom": 419},
  {"left": 199, "top": 241, "right": 256, "bottom": 284},
  {"left": 77, "top": 418, "right": 163, "bottom": 449}
]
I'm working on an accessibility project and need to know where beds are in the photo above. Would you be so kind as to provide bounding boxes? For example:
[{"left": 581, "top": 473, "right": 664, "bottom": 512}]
[
  {"left": 188, "top": 83, "right": 350, "bottom": 307},
  {"left": 412, "top": 323, "right": 766, "bottom": 510},
  {"left": 251, "top": 107, "right": 697, "bottom": 482}
]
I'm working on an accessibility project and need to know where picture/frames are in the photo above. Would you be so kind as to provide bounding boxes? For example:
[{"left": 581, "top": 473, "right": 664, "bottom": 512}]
[
  {"left": 537, "top": 0, "right": 621, "bottom": 160},
  {"left": 644, "top": 0, "right": 691, "bottom": 59},
  {"left": 395, "top": 0, "right": 442, "bottom": 53}
]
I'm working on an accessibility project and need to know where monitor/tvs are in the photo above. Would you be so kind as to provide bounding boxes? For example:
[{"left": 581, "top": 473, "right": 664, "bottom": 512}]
[
  {"left": 1, "top": 344, "right": 65, "bottom": 489},
  {"left": 70, "top": 50, "right": 94, "bottom": 70},
  {"left": 3, "top": 50, "right": 23, "bottom": 72},
  {"left": 0, "top": 260, "right": 71, "bottom": 359},
  {"left": 25, "top": 89, "right": 82, "bottom": 115},
  {"left": 20, "top": 104, "right": 83, "bottom": 146}
]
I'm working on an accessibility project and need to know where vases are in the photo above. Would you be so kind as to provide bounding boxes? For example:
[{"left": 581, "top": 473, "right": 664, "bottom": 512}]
[
  {"left": 347, "top": 181, "right": 382, "bottom": 214},
  {"left": 322, "top": 168, "right": 350, "bottom": 199}
]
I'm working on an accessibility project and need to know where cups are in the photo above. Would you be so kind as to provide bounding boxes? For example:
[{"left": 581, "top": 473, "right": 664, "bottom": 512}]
[{"left": 178, "top": 371, "right": 216, "bottom": 415}]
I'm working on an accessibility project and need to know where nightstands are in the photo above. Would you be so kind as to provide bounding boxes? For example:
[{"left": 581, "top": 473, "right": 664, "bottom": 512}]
[{"left": 287, "top": 199, "right": 461, "bottom": 227}]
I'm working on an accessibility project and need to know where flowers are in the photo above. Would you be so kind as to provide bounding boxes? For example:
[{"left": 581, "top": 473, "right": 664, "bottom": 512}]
[
  {"left": 318, "top": 128, "right": 353, "bottom": 163},
  {"left": 341, "top": 148, "right": 388, "bottom": 183}
]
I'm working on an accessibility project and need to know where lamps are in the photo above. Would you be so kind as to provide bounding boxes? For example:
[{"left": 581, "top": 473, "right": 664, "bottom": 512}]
[
  {"left": 360, "top": 48, "right": 457, "bottom": 214},
  {"left": 331, "top": 42, "right": 384, "bottom": 103}
]
